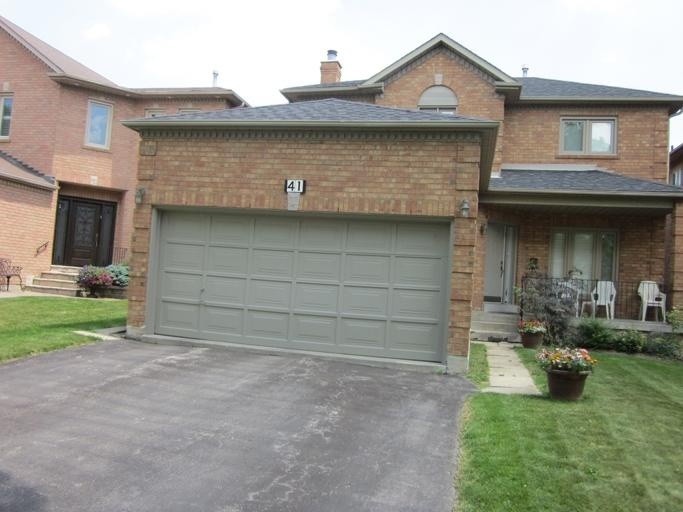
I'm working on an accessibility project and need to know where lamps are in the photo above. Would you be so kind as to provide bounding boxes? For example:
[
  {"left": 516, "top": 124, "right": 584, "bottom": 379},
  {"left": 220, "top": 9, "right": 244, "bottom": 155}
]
[
  {"left": 135, "top": 187, "right": 146, "bottom": 204},
  {"left": 460, "top": 199, "right": 470, "bottom": 217}
]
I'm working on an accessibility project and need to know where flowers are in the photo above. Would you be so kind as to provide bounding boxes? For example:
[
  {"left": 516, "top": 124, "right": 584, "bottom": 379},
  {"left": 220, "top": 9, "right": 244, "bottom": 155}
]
[
  {"left": 533, "top": 347, "right": 599, "bottom": 373},
  {"left": 76, "top": 264, "right": 113, "bottom": 287},
  {"left": 516, "top": 320, "right": 548, "bottom": 333}
]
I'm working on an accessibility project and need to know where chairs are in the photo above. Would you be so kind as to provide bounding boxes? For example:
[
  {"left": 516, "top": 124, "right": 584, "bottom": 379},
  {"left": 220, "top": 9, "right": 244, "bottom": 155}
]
[
  {"left": 637, "top": 281, "right": 667, "bottom": 323},
  {"left": 0, "top": 258, "right": 22, "bottom": 291},
  {"left": 581, "top": 281, "right": 617, "bottom": 319},
  {"left": 554, "top": 281, "right": 581, "bottom": 317}
]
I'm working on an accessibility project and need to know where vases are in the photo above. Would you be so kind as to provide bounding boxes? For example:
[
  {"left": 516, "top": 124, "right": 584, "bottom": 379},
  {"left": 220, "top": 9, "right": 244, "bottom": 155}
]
[
  {"left": 521, "top": 333, "right": 543, "bottom": 348},
  {"left": 87, "top": 286, "right": 98, "bottom": 298},
  {"left": 548, "top": 371, "right": 588, "bottom": 400}
]
